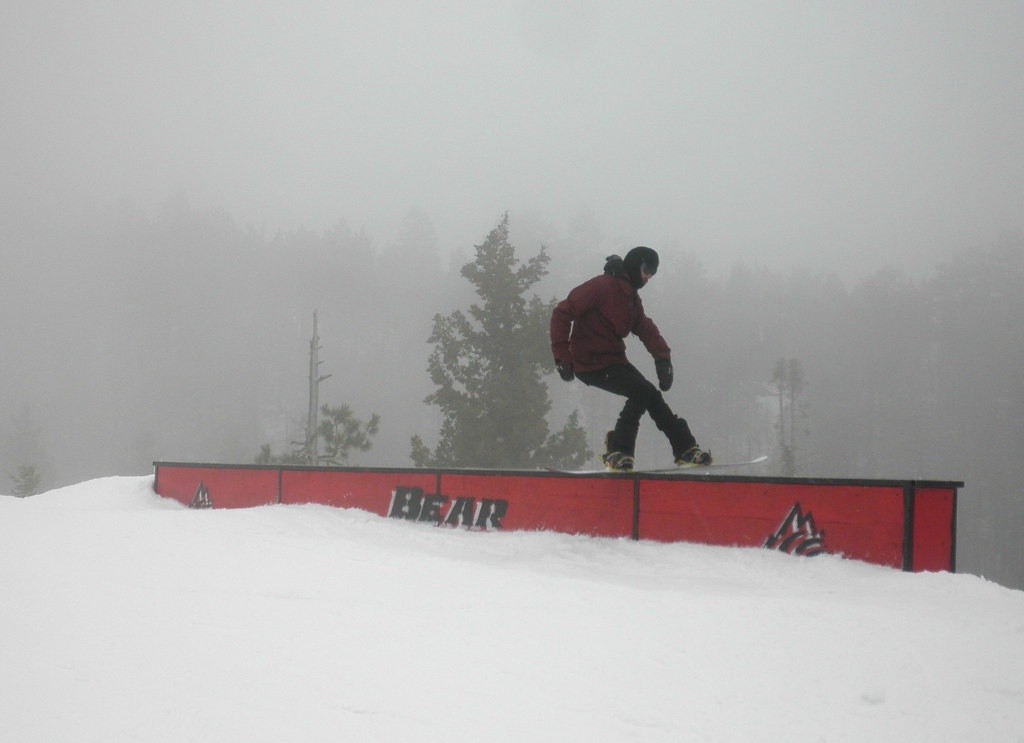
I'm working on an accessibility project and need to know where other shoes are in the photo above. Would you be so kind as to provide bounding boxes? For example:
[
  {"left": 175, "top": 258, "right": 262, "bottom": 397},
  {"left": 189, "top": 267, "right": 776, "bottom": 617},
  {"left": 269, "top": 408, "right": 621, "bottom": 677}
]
[
  {"left": 601, "top": 432, "right": 635, "bottom": 472},
  {"left": 671, "top": 443, "right": 717, "bottom": 467}
]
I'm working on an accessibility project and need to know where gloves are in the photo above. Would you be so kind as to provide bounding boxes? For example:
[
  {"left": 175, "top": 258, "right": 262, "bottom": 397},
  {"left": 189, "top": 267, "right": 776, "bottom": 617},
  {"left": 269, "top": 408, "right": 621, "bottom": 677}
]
[
  {"left": 656, "top": 359, "right": 674, "bottom": 391},
  {"left": 555, "top": 359, "right": 574, "bottom": 381}
]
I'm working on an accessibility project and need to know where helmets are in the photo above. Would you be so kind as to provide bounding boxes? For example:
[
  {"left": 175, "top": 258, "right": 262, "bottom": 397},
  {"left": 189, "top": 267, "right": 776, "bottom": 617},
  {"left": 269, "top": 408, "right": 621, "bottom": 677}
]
[{"left": 624, "top": 246, "right": 659, "bottom": 275}]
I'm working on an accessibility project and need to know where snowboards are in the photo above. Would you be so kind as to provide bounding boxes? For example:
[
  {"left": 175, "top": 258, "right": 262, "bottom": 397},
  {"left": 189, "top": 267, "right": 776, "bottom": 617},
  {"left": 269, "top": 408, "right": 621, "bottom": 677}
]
[{"left": 545, "top": 455, "right": 772, "bottom": 477}]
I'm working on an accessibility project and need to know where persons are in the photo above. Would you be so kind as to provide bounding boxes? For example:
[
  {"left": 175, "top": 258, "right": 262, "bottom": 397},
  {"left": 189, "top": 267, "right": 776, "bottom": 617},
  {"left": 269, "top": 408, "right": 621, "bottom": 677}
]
[{"left": 551, "top": 243, "right": 714, "bottom": 471}]
